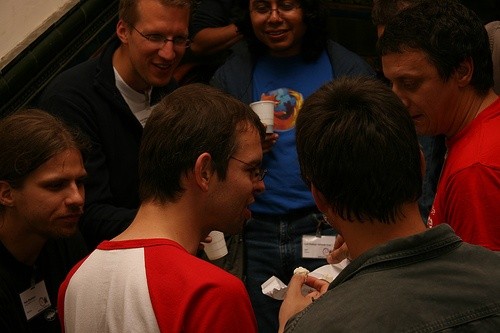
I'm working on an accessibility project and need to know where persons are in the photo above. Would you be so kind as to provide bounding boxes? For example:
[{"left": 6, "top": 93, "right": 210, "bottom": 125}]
[
  {"left": 379, "top": 0, "right": 500, "bottom": 250},
  {"left": 278, "top": 76, "right": 500, "bottom": 333},
  {"left": 208, "top": 0, "right": 379, "bottom": 333},
  {"left": 0, "top": 84, "right": 265, "bottom": 333},
  {"left": 42, "top": 0, "right": 191, "bottom": 291}
]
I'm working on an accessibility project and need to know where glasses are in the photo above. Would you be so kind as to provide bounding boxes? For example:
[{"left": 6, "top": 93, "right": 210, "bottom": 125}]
[
  {"left": 230, "top": 156, "right": 267, "bottom": 180},
  {"left": 125, "top": 21, "right": 191, "bottom": 48},
  {"left": 251, "top": 2, "right": 301, "bottom": 13}
]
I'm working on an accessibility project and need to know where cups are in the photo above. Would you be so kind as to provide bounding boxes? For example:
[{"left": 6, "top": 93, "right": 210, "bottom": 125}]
[
  {"left": 249, "top": 100, "right": 275, "bottom": 134},
  {"left": 200, "top": 230, "right": 229, "bottom": 260}
]
